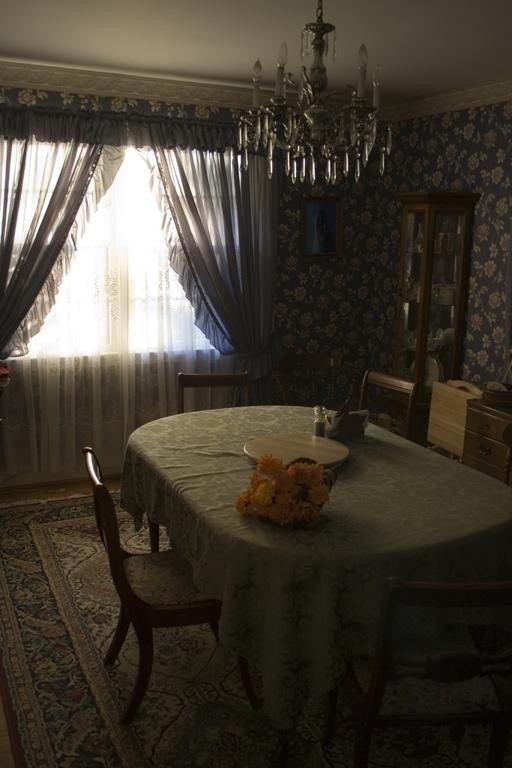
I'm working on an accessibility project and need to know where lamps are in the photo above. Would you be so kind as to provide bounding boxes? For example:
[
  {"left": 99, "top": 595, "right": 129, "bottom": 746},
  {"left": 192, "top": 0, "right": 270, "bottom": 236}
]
[{"left": 227, "top": 0, "right": 395, "bottom": 186}]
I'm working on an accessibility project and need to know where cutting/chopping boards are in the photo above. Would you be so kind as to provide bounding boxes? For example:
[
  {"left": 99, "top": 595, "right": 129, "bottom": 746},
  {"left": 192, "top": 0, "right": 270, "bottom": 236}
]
[{"left": 243, "top": 434, "right": 349, "bottom": 468}]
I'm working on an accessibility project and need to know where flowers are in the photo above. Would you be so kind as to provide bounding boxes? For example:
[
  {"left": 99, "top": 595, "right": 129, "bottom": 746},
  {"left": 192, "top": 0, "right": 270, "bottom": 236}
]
[{"left": 234, "top": 452, "right": 328, "bottom": 529}]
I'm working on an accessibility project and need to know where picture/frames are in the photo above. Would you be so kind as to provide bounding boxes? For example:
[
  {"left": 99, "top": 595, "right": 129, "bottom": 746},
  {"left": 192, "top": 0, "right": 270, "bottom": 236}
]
[{"left": 301, "top": 196, "right": 341, "bottom": 259}]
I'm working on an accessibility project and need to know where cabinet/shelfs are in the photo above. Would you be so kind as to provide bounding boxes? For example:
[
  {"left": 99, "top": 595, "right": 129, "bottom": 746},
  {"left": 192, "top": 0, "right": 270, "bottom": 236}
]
[
  {"left": 394, "top": 190, "right": 480, "bottom": 383},
  {"left": 177, "top": 371, "right": 250, "bottom": 412},
  {"left": 426, "top": 379, "right": 482, "bottom": 463},
  {"left": 462, "top": 397, "right": 512, "bottom": 485}
]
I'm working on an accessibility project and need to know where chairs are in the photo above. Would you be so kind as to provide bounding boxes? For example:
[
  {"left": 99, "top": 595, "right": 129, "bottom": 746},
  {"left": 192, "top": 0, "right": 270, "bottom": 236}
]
[
  {"left": 277, "top": 352, "right": 339, "bottom": 411},
  {"left": 81, "top": 446, "right": 262, "bottom": 726},
  {"left": 322, "top": 576, "right": 512, "bottom": 768},
  {"left": 358, "top": 368, "right": 419, "bottom": 440}
]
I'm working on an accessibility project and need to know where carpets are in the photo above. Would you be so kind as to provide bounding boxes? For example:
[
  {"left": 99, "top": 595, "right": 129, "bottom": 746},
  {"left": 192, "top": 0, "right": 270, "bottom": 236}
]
[{"left": 0, "top": 489, "right": 511, "bottom": 768}]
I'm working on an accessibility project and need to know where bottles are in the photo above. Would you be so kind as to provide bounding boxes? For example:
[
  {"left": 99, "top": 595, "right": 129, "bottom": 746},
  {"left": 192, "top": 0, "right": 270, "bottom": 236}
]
[{"left": 314, "top": 406, "right": 326, "bottom": 436}]
